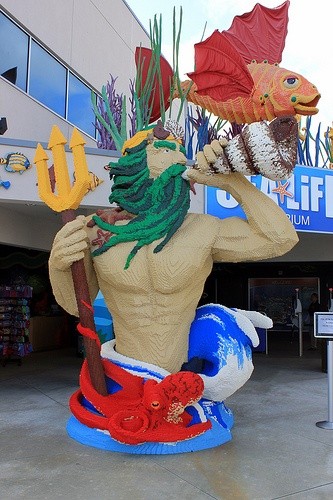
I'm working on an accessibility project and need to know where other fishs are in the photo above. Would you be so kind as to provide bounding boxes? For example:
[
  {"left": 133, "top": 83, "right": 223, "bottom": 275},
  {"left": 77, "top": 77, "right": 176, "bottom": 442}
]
[{"left": 134, "top": 0, "right": 322, "bottom": 129}]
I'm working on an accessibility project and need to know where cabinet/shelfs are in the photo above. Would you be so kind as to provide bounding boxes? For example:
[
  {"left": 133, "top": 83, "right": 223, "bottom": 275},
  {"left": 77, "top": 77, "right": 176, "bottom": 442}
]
[{"left": 0, "top": 286, "right": 32, "bottom": 366}]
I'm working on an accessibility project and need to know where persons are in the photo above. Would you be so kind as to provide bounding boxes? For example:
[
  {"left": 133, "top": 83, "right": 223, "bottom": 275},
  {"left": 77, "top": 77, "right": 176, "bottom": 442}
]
[{"left": 307, "top": 293, "right": 323, "bottom": 353}]
